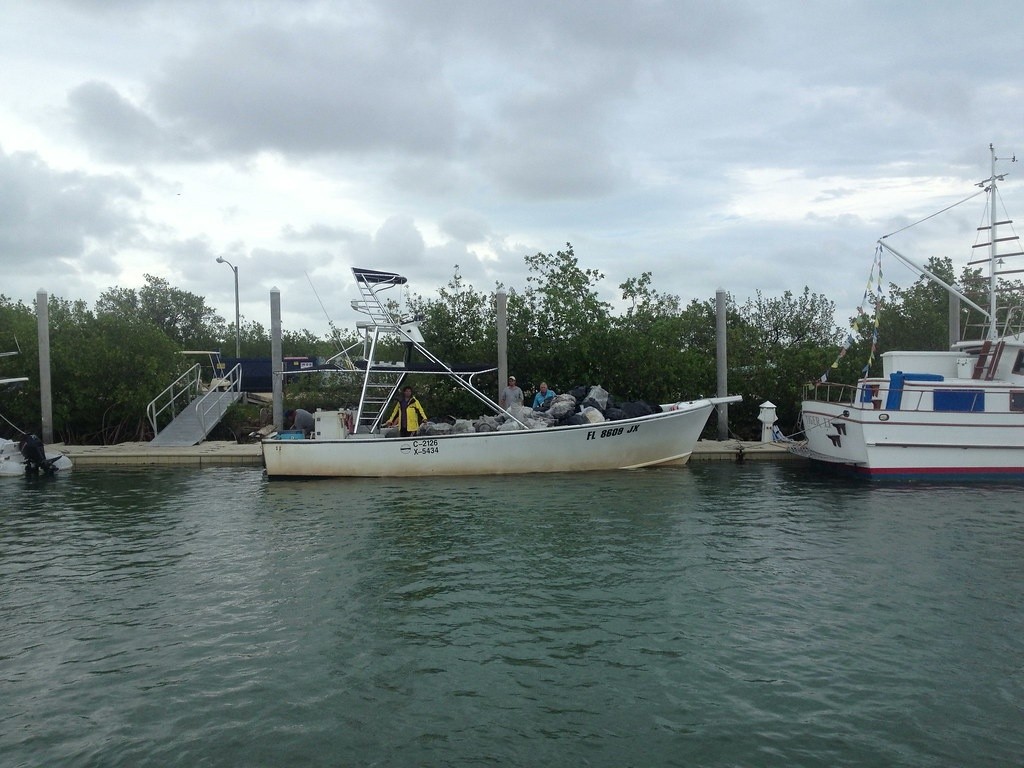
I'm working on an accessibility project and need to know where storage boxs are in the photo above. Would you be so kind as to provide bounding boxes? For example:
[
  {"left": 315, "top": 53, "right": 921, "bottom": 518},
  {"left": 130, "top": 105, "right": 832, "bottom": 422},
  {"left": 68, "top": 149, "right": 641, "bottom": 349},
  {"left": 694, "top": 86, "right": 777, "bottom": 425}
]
[{"left": 277, "top": 430, "right": 305, "bottom": 440}]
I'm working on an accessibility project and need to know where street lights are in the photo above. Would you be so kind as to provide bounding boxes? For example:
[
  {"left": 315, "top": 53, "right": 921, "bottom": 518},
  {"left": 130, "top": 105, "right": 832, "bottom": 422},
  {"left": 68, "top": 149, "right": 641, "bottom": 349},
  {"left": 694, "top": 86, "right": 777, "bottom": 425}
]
[{"left": 216, "top": 256, "right": 240, "bottom": 358}]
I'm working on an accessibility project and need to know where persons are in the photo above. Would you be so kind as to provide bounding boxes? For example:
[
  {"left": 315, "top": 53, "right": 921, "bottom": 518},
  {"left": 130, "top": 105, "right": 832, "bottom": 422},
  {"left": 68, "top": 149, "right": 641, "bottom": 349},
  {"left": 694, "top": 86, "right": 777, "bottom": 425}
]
[
  {"left": 285, "top": 407, "right": 315, "bottom": 436},
  {"left": 499, "top": 376, "right": 524, "bottom": 410},
  {"left": 386, "top": 386, "right": 428, "bottom": 436},
  {"left": 532, "top": 382, "right": 557, "bottom": 412}
]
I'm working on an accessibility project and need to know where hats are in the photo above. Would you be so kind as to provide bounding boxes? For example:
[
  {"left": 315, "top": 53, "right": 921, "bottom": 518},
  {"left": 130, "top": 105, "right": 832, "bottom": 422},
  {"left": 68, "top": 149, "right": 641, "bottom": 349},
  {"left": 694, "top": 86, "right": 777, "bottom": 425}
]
[{"left": 509, "top": 376, "right": 515, "bottom": 380}]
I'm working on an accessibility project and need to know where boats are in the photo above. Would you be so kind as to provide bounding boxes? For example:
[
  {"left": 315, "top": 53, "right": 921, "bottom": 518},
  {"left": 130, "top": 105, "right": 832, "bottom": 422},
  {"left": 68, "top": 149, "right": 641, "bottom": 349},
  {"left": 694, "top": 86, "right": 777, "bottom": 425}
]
[
  {"left": 800, "top": 143, "right": 1024, "bottom": 487},
  {"left": 0, "top": 436, "right": 73, "bottom": 475},
  {"left": 263, "top": 395, "right": 748, "bottom": 477}
]
